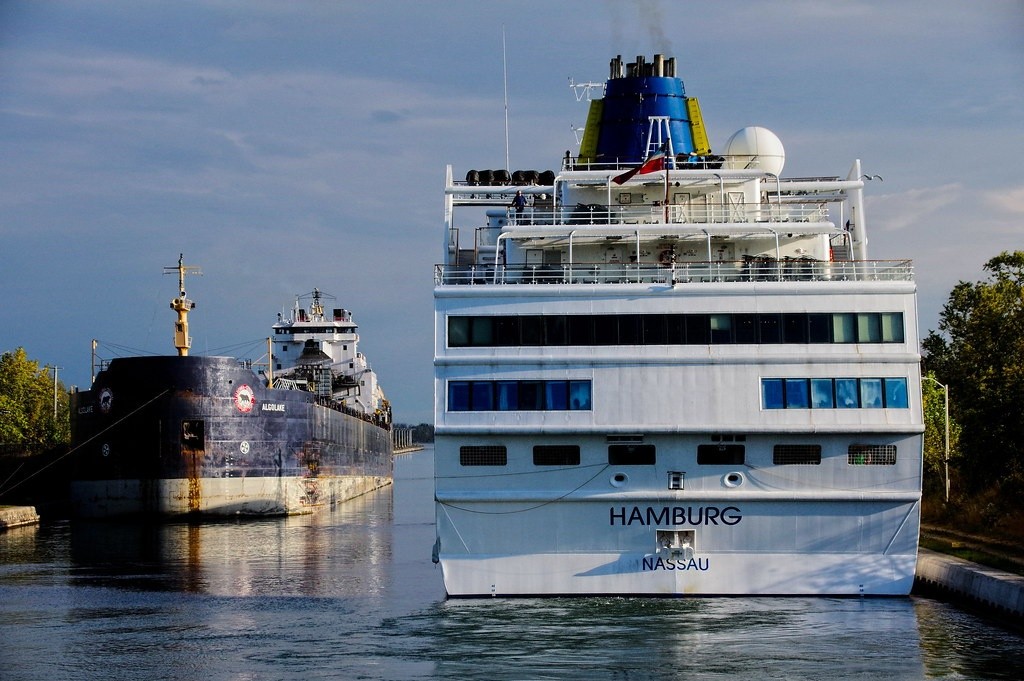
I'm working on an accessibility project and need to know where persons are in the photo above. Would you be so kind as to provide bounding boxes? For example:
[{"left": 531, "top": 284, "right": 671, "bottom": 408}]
[{"left": 511, "top": 191, "right": 528, "bottom": 226}]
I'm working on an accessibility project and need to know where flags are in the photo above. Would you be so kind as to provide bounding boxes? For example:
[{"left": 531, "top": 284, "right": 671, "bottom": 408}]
[{"left": 612, "top": 139, "right": 668, "bottom": 185}]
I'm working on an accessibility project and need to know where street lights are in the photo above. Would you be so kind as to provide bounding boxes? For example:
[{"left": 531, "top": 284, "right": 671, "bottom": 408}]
[{"left": 922, "top": 377, "right": 950, "bottom": 502}]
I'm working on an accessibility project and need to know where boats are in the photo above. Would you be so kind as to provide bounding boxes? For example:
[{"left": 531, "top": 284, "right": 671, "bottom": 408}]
[
  {"left": 68, "top": 253, "right": 393, "bottom": 520},
  {"left": 434, "top": 31, "right": 925, "bottom": 596}
]
